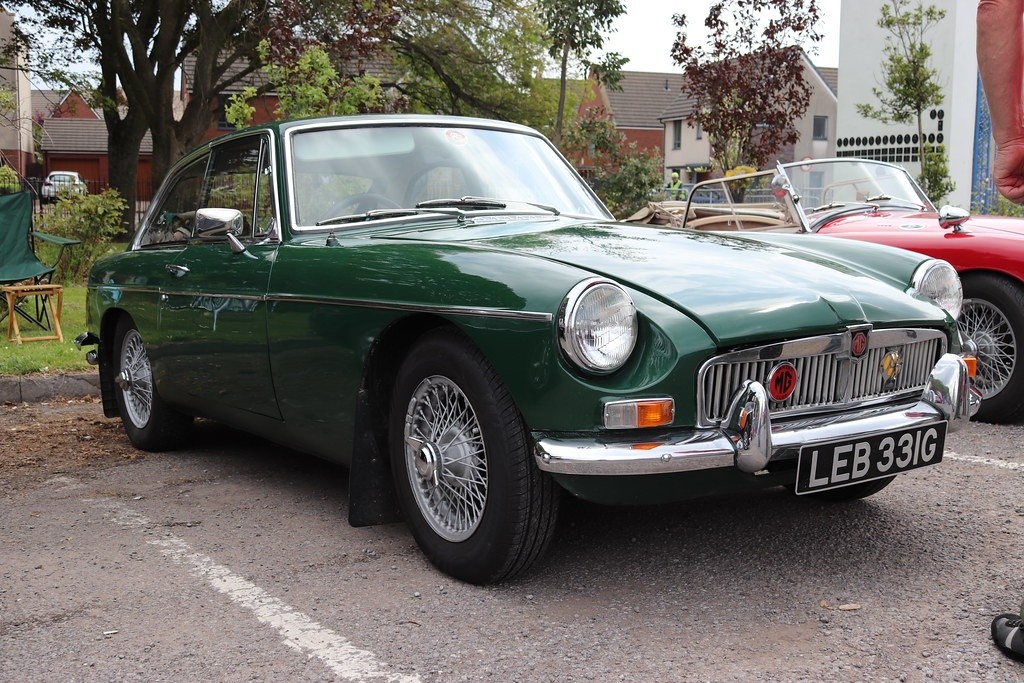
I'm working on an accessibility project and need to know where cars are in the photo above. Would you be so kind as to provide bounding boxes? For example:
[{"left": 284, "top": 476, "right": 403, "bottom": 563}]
[
  {"left": 75, "top": 115, "right": 973, "bottom": 587},
  {"left": 665, "top": 183, "right": 728, "bottom": 204},
  {"left": 677, "top": 155, "right": 1024, "bottom": 426},
  {"left": 41, "top": 171, "right": 91, "bottom": 205}
]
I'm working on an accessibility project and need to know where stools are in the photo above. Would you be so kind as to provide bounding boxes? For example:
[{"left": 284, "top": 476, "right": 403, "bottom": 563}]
[{"left": 1, "top": 284, "right": 63, "bottom": 344}]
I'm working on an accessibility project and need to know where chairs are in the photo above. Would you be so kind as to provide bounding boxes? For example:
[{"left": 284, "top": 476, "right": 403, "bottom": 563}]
[{"left": 0, "top": 190, "right": 82, "bottom": 333}]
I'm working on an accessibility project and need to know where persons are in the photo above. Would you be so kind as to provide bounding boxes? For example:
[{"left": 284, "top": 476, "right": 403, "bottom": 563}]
[
  {"left": 976, "top": 0, "right": 1024, "bottom": 666},
  {"left": 667, "top": 172, "right": 685, "bottom": 200}
]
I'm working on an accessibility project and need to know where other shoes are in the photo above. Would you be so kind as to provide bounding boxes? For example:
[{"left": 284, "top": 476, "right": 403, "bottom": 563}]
[{"left": 991, "top": 613, "right": 1024, "bottom": 664}]
